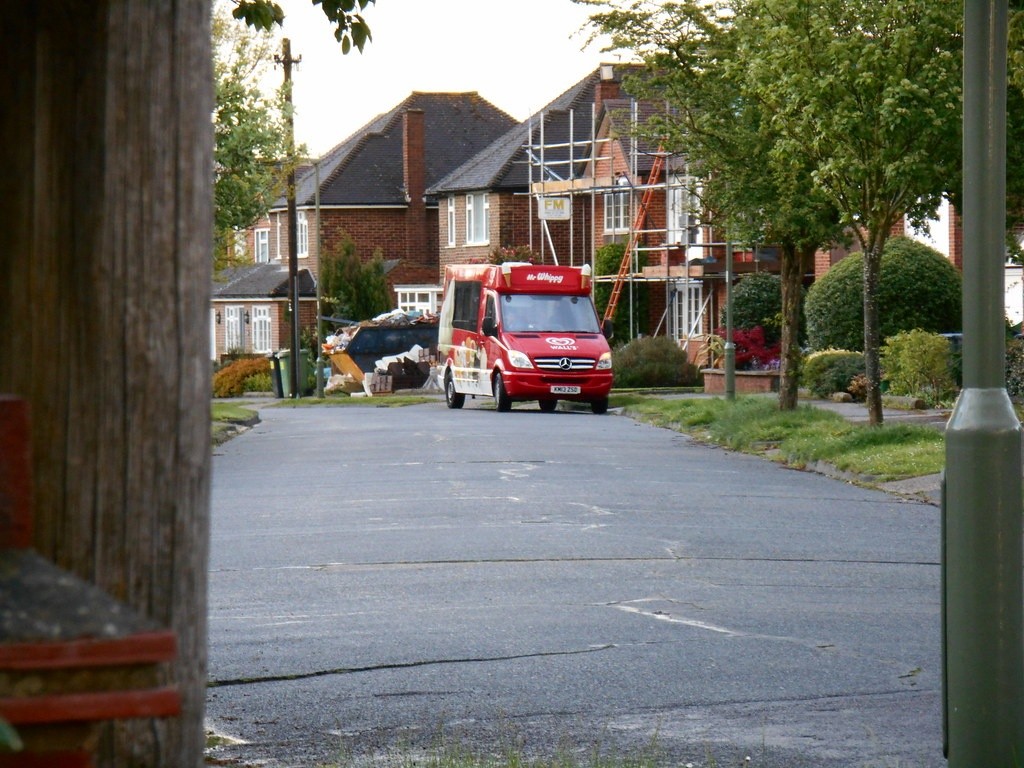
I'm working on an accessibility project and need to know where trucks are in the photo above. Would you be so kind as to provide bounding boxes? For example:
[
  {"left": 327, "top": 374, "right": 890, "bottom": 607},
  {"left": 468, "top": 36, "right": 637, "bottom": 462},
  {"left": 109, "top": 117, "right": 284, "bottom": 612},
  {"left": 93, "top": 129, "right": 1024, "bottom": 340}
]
[{"left": 436, "top": 261, "right": 614, "bottom": 415}]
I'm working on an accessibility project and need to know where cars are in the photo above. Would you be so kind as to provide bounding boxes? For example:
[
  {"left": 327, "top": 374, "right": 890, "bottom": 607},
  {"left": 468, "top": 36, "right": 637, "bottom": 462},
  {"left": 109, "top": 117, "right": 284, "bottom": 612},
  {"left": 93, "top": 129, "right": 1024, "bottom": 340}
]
[
  {"left": 940, "top": 333, "right": 962, "bottom": 390},
  {"left": 1013, "top": 334, "right": 1024, "bottom": 342}
]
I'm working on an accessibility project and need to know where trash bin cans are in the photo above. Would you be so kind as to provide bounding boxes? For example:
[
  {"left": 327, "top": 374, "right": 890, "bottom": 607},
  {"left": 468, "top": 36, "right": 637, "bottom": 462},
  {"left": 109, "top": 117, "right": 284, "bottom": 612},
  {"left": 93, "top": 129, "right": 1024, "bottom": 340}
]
[
  {"left": 275, "top": 350, "right": 308, "bottom": 399},
  {"left": 266, "top": 352, "right": 283, "bottom": 399}
]
